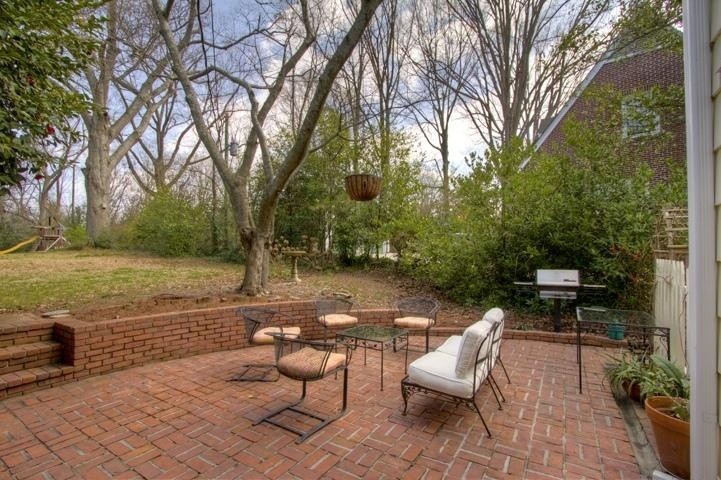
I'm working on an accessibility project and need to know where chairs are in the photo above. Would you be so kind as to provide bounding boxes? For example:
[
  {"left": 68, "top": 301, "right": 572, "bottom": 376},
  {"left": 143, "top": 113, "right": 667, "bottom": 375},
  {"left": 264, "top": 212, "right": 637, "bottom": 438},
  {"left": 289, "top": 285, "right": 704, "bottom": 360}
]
[
  {"left": 401, "top": 321, "right": 503, "bottom": 440},
  {"left": 226, "top": 306, "right": 302, "bottom": 382},
  {"left": 311, "top": 296, "right": 362, "bottom": 350},
  {"left": 435, "top": 307, "right": 511, "bottom": 402},
  {"left": 392, "top": 297, "right": 440, "bottom": 354},
  {"left": 252, "top": 331, "right": 352, "bottom": 445}
]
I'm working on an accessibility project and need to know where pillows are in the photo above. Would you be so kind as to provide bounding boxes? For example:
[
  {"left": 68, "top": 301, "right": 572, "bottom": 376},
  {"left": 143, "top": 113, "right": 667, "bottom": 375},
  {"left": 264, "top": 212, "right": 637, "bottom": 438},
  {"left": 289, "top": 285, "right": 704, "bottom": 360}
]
[{"left": 453, "top": 307, "right": 506, "bottom": 378}]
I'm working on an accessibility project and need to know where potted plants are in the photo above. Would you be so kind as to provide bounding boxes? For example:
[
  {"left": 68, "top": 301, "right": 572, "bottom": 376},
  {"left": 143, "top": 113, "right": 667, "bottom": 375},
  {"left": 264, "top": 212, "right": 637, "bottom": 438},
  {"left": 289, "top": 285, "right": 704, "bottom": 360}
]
[
  {"left": 639, "top": 354, "right": 691, "bottom": 480},
  {"left": 599, "top": 349, "right": 647, "bottom": 401}
]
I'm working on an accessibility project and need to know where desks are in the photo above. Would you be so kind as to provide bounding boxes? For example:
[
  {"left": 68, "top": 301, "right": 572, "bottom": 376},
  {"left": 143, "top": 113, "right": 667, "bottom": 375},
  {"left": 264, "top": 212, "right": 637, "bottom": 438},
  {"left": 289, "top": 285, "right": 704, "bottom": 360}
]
[{"left": 573, "top": 306, "right": 671, "bottom": 396}]
